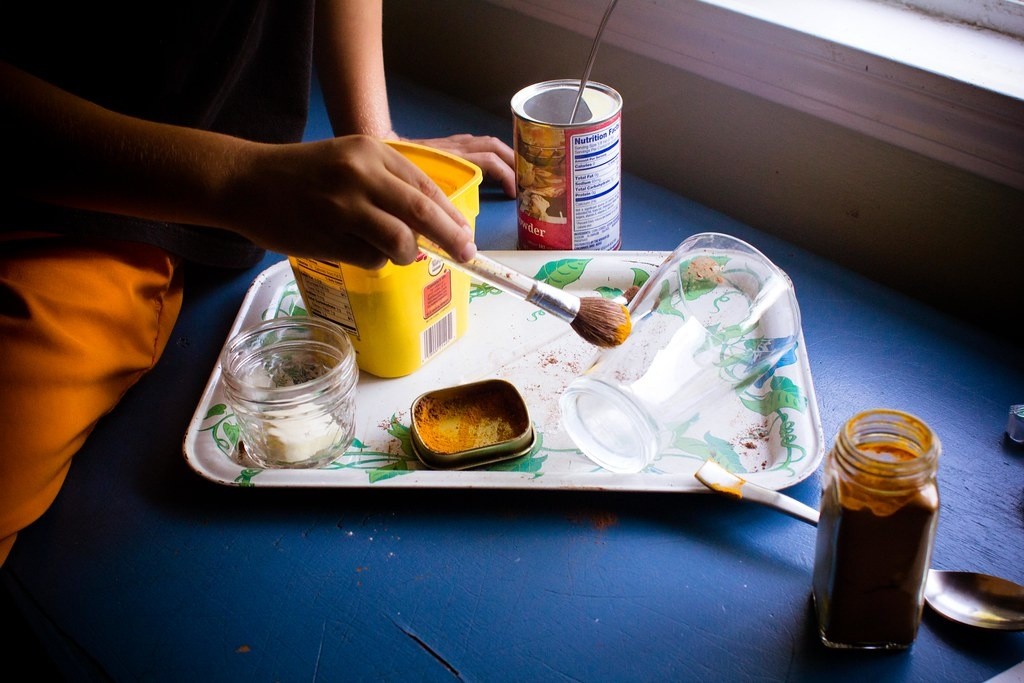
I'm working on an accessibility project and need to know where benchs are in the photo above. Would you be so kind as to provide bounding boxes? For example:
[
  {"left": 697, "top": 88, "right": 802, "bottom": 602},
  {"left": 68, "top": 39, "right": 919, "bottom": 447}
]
[{"left": 0, "top": 46, "right": 1024, "bottom": 681}]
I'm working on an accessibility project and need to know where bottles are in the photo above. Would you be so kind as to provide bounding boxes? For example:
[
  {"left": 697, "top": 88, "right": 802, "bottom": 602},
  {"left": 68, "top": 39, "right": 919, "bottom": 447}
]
[{"left": 810, "top": 409, "right": 942, "bottom": 656}]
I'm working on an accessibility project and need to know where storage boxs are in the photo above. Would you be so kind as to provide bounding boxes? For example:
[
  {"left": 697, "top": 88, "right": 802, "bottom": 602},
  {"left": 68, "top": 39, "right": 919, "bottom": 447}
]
[{"left": 287, "top": 140, "right": 484, "bottom": 382}]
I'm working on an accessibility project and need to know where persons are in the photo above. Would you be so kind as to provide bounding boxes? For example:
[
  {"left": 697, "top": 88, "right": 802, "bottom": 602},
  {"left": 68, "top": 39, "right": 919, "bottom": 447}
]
[{"left": 0, "top": 1, "right": 515, "bottom": 573}]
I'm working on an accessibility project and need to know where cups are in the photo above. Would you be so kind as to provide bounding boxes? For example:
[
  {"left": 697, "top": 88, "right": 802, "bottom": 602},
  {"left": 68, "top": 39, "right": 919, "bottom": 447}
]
[
  {"left": 557, "top": 233, "right": 800, "bottom": 476},
  {"left": 223, "top": 315, "right": 360, "bottom": 469}
]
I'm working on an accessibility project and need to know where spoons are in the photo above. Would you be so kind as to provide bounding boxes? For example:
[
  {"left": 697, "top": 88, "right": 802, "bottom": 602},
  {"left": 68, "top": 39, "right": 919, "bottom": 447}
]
[{"left": 696, "top": 456, "right": 1024, "bottom": 630}]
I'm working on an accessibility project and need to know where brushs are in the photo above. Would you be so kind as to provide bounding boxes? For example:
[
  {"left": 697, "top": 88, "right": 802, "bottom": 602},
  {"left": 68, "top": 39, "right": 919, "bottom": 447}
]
[{"left": 415, "top": 235, "right": 632, "bottom": 350}]
